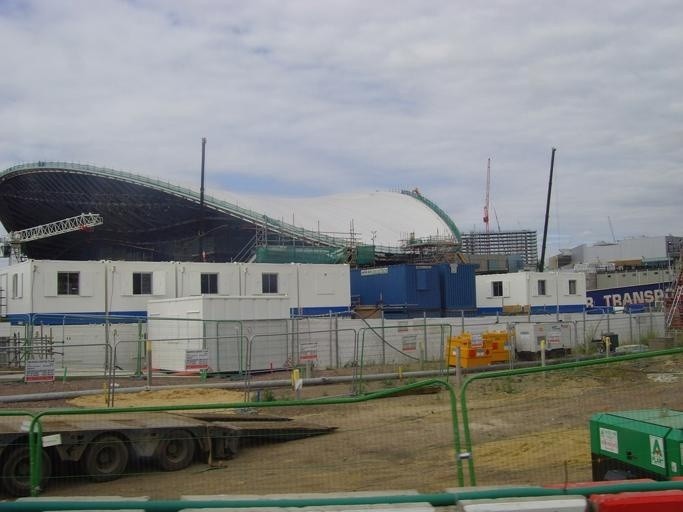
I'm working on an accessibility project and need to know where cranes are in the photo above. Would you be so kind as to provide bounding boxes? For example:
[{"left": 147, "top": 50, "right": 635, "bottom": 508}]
[
  {"left": 481, "top": 155, "right": 495, "bottom": 233},
  {"left": 0, "top": 212, "right": 107, "bottom": 267}
]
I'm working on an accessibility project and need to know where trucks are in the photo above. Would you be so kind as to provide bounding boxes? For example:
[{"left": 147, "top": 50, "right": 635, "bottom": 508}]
[{"left": 0, "top": 410, "right": 339, "bottom": 495}]
